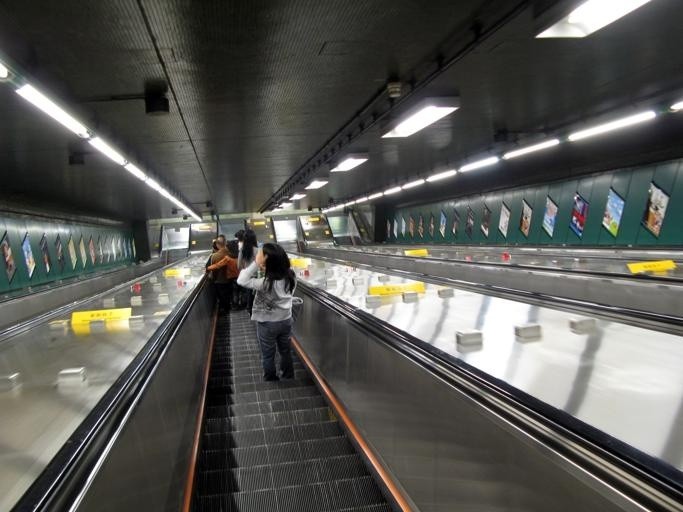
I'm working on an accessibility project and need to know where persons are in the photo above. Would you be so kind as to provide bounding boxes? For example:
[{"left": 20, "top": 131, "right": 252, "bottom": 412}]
[
  {"left": 236, "top": 243, "right": 297, "bottom": 381},
  {"left": 206, "top": 229, "right": 259, "bottom": 314}
]
